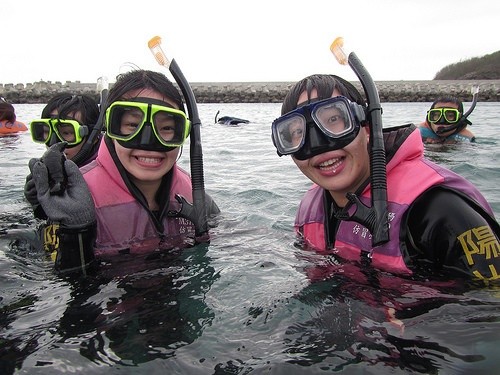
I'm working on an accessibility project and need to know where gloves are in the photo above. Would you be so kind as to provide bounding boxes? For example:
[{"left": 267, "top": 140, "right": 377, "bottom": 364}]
[
  {"left": 29, "top": 157, "right": 96, "bottom": 229},
  {"left": 24, "top": 172, "right": 39, "bottom": 208}
]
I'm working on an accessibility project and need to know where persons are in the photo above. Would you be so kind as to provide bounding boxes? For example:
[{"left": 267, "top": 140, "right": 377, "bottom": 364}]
[
  {"left": 273, "top": 74, "right": 500, "bottom": 288},
  {"left": 24, "top": 92, "right": 108, "bottom": 221},
  {"left": 30, "top": 70, "right": 221, "bottom": 279},
  {"left": 418, "top": 93, "right": 476, "bottom": 146},
  {"left": 0, "top": 94, "right": 28, "bottom": 134}
]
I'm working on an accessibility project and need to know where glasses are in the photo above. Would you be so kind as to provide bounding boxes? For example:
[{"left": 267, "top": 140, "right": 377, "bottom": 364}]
[
  {"left": 104, "top": 101, "right": 193, "bottom": 147},
  {"left": 29, "top": 118, "right": 94, "bottom": 146},
  {"left": 427, "top": 108, "right": 462, "bottom": 123},
  {"left": 271, "top": 95, "right": 369, "bottom": 155}
]
